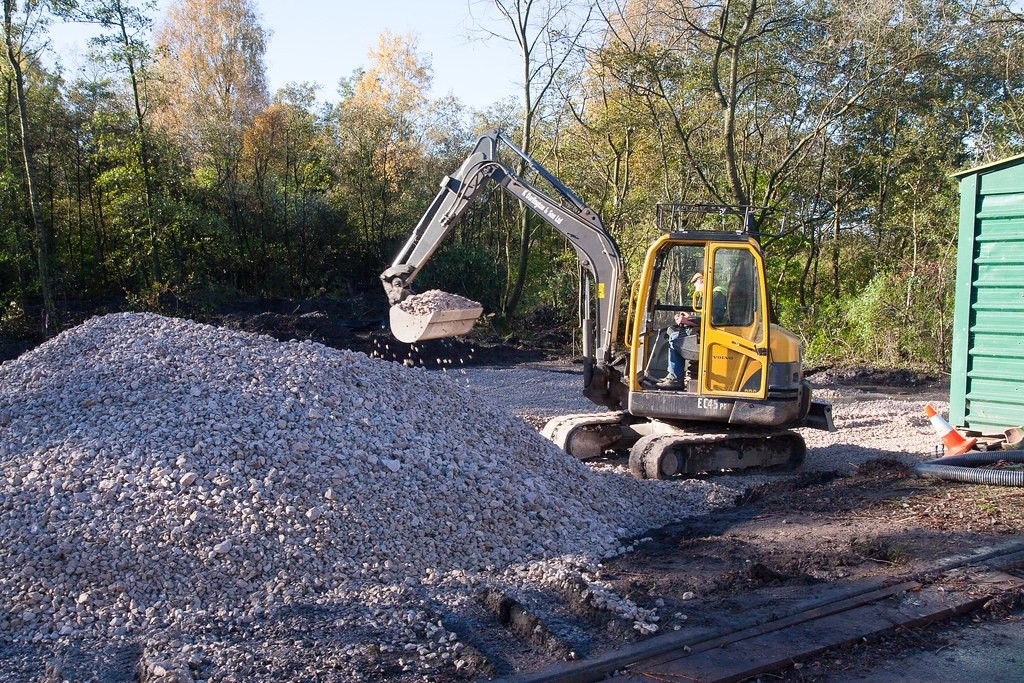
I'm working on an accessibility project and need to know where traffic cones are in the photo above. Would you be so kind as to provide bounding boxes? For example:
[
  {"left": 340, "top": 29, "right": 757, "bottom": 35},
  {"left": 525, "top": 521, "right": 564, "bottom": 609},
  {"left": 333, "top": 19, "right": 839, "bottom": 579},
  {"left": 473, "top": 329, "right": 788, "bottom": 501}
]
[{"left": 923, "top": 404, "right": 977, "bottom": 457}]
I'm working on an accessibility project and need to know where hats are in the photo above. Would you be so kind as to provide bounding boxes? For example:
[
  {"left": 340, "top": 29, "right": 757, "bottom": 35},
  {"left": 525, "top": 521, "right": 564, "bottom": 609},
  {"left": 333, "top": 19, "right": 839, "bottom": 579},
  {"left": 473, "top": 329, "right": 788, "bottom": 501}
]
[{"left": 690, "top": 270, "right": 703, "bottom": 283}]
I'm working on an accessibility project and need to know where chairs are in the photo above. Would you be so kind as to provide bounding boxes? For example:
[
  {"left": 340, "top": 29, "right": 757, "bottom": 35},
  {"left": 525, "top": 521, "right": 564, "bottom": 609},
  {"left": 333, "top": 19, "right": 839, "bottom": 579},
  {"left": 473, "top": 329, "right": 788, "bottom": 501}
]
[{"left": 669, "top": 258, "right": 750, "bottom": 360}]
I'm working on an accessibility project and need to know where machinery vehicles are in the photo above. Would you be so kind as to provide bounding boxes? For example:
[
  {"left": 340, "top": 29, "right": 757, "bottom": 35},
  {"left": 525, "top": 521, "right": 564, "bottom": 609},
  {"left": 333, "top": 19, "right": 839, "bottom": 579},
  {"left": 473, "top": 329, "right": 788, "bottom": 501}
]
[{"left": 379, "top": 127, "right": 839, "bottom": 480}]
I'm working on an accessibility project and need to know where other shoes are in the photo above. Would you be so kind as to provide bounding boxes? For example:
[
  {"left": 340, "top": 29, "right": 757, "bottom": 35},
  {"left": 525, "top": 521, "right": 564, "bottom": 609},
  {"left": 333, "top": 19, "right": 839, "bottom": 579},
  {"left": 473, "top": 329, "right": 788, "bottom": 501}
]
[{"left": 656, "top": 376, "right": 684, "bottom": 391}]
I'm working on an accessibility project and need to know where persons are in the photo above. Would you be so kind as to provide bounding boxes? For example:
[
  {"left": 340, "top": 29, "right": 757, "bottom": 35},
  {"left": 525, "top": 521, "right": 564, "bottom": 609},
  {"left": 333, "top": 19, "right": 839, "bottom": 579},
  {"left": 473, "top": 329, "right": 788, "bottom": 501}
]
[{"left": 656, "top": 273, "right": 728, "bottom": 390}]
